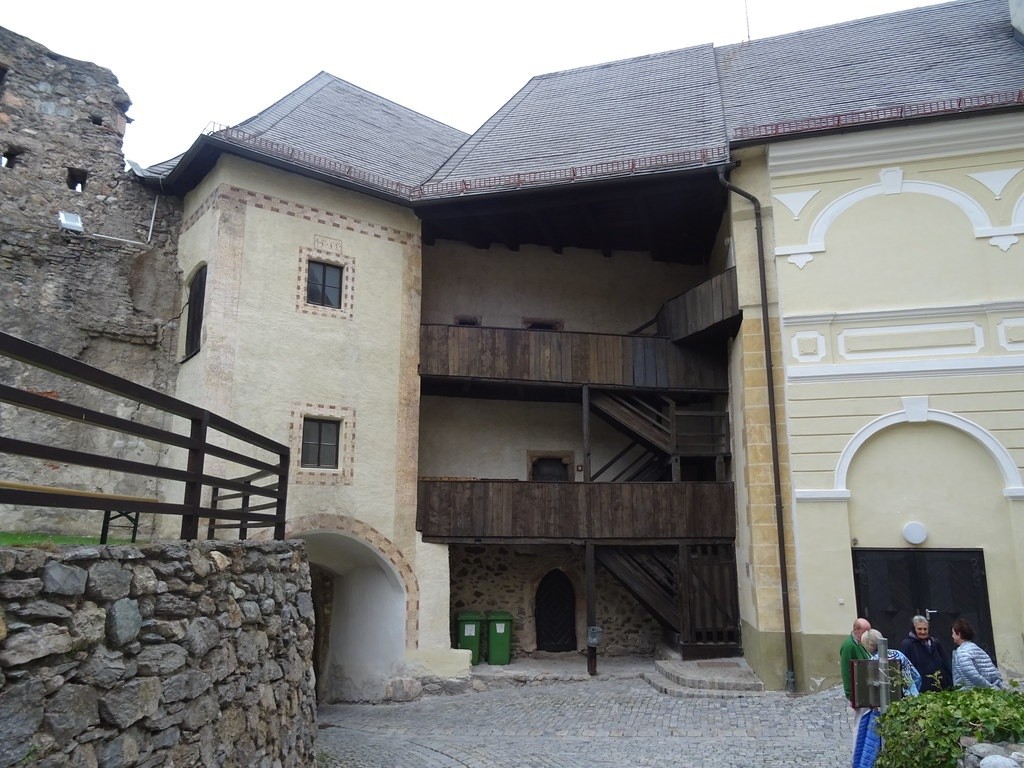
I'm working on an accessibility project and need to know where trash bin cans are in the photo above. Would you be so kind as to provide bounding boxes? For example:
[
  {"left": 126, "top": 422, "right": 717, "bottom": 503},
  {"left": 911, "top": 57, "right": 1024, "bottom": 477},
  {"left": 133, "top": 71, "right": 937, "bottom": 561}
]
[
  {"left": 484, "top": 610, "right": 514, "bottom": 666},
  {"left": 456, "top": 610, "right": 483, "bottom": 667}
]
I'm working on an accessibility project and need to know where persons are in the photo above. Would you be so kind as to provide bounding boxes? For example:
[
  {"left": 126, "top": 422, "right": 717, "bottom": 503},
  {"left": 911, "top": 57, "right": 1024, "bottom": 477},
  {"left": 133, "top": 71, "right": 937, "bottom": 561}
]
[
  {"left": 861, "top": 629, "right": 922, "bottom": 711},
  {"left": 899, "top": 615, "right": 951, "bottom": 692},
  {"left": 952, "top": 623, "right": 1005, "bottom": 693},
  {"left": 840, "top": 618, "right": 878, "bottom": 768}
]
